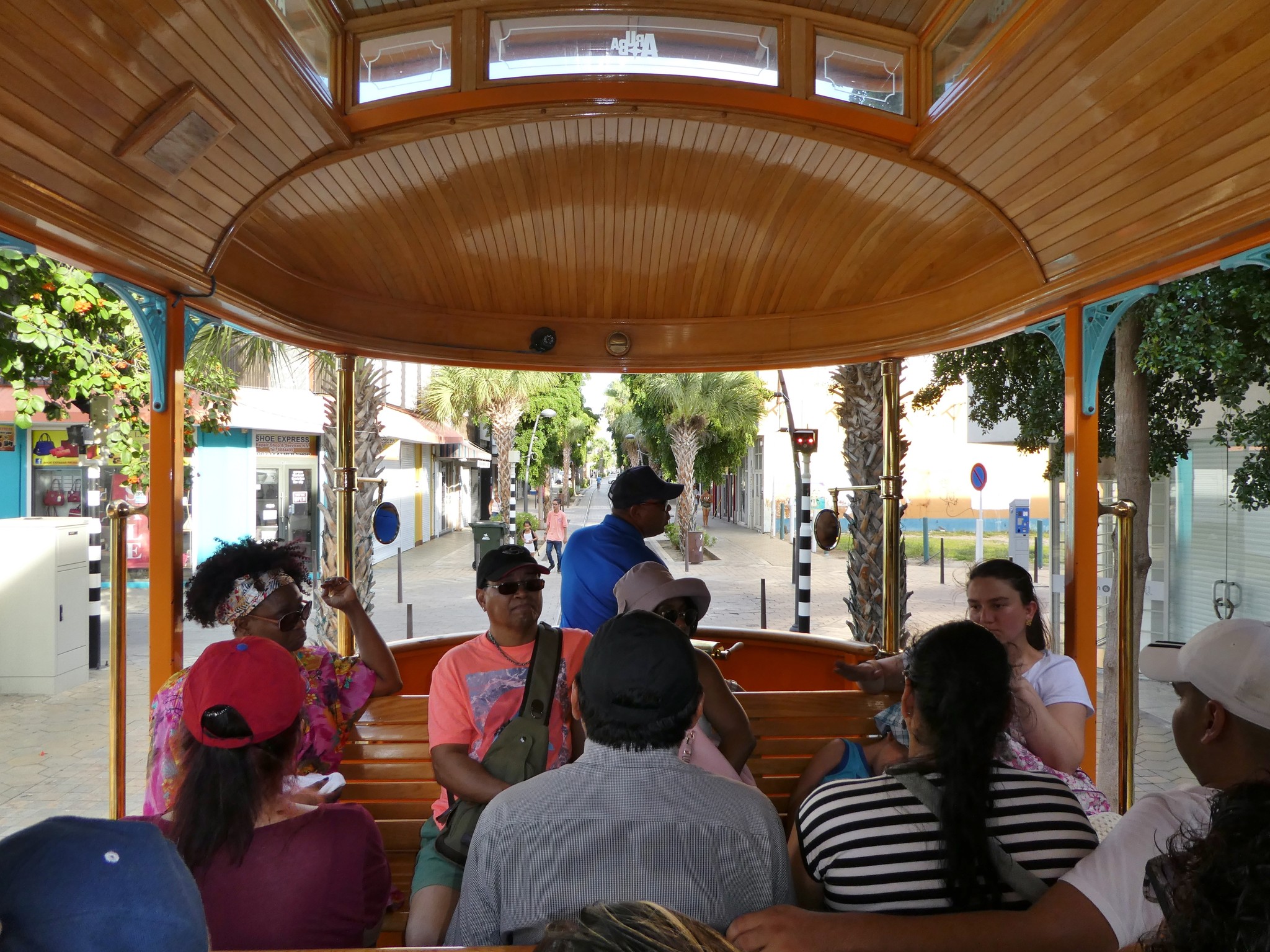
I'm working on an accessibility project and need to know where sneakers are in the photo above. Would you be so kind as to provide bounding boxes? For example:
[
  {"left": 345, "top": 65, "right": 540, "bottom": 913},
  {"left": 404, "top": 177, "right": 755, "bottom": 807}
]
[
  {"left": 557, "top": 568, "right": 561, "bottom": 573},
  {"left": 548, "top": 565, "right": 555, "bottom": 572}
]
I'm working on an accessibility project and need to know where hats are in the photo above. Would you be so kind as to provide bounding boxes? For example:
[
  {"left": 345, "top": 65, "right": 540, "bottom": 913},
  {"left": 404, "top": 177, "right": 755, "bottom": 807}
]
[
  {"left": 612, "top": 561, "right": 711, "bottom": 616},
  {"left": 580, "top": 610, "right": 697, "bottom": 721},
  {"left": 1137, "top": 619, "right": 1270, "bottom": 729},
  {"left": 182, "top": 636, "right": 306, "bottom": 749},
  {"left": 607, "top": 466, "right": 683, "bottom": 507},
  {"left": 477, "top": 545, "right": 550, "bottom": 589},
  {"left": 0, "top": 815, "right": 209, "bottom": 951},
  {"left": 874, "top": 702, "right": 910, "bottom": 746}
]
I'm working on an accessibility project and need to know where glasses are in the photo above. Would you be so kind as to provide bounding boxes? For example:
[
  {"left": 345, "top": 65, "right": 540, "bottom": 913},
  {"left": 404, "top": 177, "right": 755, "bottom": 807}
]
[
  {"left": 552, "top": 503, "right": 560, "bottom": 506},
  {"left": 640, "top": 500, "right": 669, "bottom": 512},
  {"left": 693, "top": 486, "right": 696, "bottom": 487},
  {"left": 1142, "top": 852, "right": 1190, "bottom": 952},
  {"left": 247, "top": 600, "right": 312, "bottom": 631},
  {"left": 483, "top": 578, "right": 545, "bottom": 595},
  {"left": 655, "top": 606, "right": 698, "bottom": 627}
]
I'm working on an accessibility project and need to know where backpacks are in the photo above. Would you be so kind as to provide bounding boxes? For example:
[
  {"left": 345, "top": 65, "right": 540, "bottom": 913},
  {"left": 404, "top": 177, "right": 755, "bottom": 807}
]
[{"left": 522, "top": 529, "right": 538, "bottom": 551}]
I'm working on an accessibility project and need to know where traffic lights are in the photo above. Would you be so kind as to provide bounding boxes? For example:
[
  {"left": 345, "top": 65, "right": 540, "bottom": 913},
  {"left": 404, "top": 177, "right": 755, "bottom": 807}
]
[{"left": 793, "top": 430, "right": 816, "bottom": 453}]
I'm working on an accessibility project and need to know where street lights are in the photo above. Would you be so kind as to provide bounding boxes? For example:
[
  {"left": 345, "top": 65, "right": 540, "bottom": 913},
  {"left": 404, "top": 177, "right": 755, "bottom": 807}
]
[
  {"left": 523, "top": 408, "right": 557, "bottom": 513},
  {"left": 625, "top": 434, "right": 644, "bottom": 466},
  {"left": 597, "top": 458, "right": 603, "bottom": 478}
]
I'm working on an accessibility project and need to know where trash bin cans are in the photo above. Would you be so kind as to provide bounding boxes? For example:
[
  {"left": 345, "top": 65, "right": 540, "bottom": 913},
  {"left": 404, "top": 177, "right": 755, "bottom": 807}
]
[
  {"left": 687, "top": 531, "right": 705, "bottom": 563},
  {"left": 469, "top": 521, "right": 510, "bottom": 571},
  {"left": 558, "top": 493, "right": 565, "bottom": 506}
]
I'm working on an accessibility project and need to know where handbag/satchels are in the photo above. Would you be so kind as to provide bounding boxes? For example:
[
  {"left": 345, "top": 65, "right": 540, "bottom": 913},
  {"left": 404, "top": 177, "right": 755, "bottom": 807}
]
[
  {"left": 678, "top": 723, "right": 757, "bottom": 788},
  {"left": 995, "top": 716, "right": 1110, "bottom": 814},
  {"left": 433, "top": 620, "right": 563, "bottom": 869}
]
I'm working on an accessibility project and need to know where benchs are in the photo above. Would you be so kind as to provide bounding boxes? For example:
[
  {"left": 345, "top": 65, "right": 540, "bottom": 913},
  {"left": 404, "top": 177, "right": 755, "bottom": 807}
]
[{"left": 299, "top": 622, "right": 902, "bottom": 944}]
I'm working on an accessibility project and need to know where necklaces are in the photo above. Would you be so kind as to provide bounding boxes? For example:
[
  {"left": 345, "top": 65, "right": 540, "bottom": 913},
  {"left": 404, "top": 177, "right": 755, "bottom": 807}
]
[{"left": 488, "top": 629, "right": 530, "bottom": 666}]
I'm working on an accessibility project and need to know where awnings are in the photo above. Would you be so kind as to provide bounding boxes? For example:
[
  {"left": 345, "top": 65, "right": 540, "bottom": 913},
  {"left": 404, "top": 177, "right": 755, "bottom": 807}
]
[
  {"left": 435, "top": 442, "right": 491, "bottom": 463},
  {"left": 0, "top": 385, "right": 464, "bottom": 445}
]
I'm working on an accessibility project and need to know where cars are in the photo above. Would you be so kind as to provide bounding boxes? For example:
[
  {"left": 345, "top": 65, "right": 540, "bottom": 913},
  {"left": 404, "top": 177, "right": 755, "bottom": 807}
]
[
  {"left": 555, "top": 474, "right": 563, "bottom": 485},
  {"left": 608, "top": 476, "right": 617, "bottom": 485}
]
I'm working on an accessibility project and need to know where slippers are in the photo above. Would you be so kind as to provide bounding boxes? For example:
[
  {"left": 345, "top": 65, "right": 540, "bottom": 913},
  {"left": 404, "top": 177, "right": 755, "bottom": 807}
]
[
  {"left": 705, "top": 525, "right": 709, "bottom": 527},
  {"left": 702, "top": 526, "right": 705, "bottom": 527}
]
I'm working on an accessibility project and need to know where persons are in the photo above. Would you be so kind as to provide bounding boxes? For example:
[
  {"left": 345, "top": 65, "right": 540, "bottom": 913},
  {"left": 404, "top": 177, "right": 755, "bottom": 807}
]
[
  {"left": 489, "top": 493, "right": 502, "bottom": 517},
  {"left": 119, "top": 636, "right": 391, "bottom": 952},
  {"left": 543, "top": 499, "right": 568, "bottom": 574},
  {"left": 404, "top": 544, "right": 593, "bottom": 948},
  {"left": 596, "top": 475, "right": 602, "bottom": 491},
  {"left": 443, "top": 606, "right": 793, "bottom": 947},
  {"left": 725, "top": 618, "right": 1270, "bottom": 952},
  {"left": 536, "top": 485, "right": 545, "bottom": 503},
  {"left": 611, "top": 561, "right": 757, "bottom": 778},
  {"left": 700, "top": 486, "right": 713, "bottom": 527},
  {"left": 790, "top": 727, "right": 910, "bottom": 828},
  {"left": 831, "top": 558, "right": 1095, "bottom": 775},
  {"left": 560, "top": 466, "right": 685, "bottom": 636},
  {"left": 142, "top": 536, "right": 403, "bottom": 817},
  {"left": 788, "top": 620, "right": 1100, "bottom": 915},
  {"left": 692, "top": 483, "right": 700, "bottom": 514},
  {"left": 521, "top": 521, "right": 538, "bottom": 558}
]
